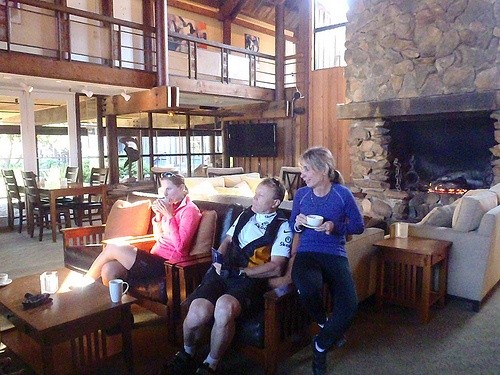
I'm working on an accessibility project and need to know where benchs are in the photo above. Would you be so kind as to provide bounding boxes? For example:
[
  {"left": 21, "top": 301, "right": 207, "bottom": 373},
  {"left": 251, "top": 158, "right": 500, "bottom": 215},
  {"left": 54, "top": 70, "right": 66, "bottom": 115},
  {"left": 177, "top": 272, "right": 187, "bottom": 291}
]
[
  {"left": 396, "top": 182, "right": 500, "bottom": 312},
  {"left": 60, "top": 172, "right": 385, "bottom": 375}
]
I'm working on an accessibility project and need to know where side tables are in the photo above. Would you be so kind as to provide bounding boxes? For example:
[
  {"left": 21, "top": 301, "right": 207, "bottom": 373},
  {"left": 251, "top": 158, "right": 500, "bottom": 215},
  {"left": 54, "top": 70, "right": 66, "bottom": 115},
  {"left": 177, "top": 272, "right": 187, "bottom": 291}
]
[{"left": 372, "top": 236, "right": 453, "bottom": 325}]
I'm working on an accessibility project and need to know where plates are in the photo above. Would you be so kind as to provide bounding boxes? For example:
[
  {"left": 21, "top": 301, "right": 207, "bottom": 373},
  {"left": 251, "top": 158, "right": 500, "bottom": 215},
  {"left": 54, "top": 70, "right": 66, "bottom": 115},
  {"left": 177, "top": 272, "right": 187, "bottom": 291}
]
[
  {"left": 304, "top": 224, "right": 320, "bottom": 229},
  {"left": 0, "top": 279, "right": 12, "bottom": 286}
]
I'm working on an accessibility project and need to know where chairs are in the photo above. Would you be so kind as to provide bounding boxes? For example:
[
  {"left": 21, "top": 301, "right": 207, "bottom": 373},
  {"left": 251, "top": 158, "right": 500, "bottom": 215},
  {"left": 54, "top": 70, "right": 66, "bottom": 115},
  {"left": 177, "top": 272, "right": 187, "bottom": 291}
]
[
  {"left": 207, "top": 167, "right": 243, "bottom": 178},
  {"left": 1, "top": 167, "right": 109, "bottom": 242},
  {"left": 279, "top": 167, "right": 307, "bottom": 201},
  {"left": 176, "top": 209, "right": 333, "bottom": 375},
  {"left": 151, "top": 167, "right": 179, "bottom": 191}
]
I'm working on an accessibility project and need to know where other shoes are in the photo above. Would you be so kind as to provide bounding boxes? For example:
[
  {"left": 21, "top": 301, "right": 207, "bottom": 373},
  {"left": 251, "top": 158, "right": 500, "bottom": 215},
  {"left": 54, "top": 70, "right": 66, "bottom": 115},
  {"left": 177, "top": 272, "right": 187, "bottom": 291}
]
[
  {"left": 169, "top": 350, "right": 193, "bottom": 373},
  {"left": 196, "top": 357, "right": 220, "bottom": 375},
  {"left": 319, "top": 319, "right": 345, "bottom": 348},
  {"left": 312, "top": 336, "right": 326, "bottom": 375},
  {"left": 106, "top": 313, "right": 135, "bottom": 335}
]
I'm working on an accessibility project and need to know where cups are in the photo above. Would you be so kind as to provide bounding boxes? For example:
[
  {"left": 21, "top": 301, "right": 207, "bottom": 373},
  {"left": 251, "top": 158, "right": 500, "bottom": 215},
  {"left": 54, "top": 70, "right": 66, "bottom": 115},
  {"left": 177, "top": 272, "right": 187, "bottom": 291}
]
[
  {"left": 109, "top": 280, "right": 129, "bottom": 303},
  {"left": 156, "top": 199, "right": 170, "bottom": 205},
  {"left": 0, "top": 274, "right": 8, "bottom": 284},
  {"left": 305, "top": 215, "right": 323, "bottom": 227}
]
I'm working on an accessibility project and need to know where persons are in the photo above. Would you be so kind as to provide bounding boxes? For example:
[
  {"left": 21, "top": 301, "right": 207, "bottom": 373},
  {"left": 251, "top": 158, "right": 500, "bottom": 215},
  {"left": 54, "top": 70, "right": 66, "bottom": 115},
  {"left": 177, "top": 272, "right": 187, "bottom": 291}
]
[
  {"left": 171, "top": 179, "right": 292, "bottom": 375},
  {"left": 290, "top": 148, "right": 364, "bottom": 375},
  {"left": 85, "top": 173, "right": 201, "bottom": 335}
]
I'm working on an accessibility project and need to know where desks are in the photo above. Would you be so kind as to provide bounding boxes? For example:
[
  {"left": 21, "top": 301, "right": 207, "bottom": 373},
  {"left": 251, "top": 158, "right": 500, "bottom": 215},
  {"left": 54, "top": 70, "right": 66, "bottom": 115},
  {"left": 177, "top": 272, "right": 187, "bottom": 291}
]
[{"left": 18, "top": 181, "right": 107, "bottom": 243}]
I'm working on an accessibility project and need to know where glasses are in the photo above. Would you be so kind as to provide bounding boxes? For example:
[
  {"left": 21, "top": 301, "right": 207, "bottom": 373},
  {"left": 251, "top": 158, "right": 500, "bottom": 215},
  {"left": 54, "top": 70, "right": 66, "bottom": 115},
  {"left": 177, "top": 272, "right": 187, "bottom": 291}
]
[
  {"left": 161, "top": 172, "right": 176, "bottom": 178},
  {"left": 270, "top": 177, "right": 281, "bottom": 195}
]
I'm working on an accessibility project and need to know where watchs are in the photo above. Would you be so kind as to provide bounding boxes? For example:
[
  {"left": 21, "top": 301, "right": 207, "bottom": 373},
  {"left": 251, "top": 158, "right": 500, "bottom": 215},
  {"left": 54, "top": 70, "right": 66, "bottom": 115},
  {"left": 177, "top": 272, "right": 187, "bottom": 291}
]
[{"left": 239, "top": 267, "right": 246, "bottom": 278}]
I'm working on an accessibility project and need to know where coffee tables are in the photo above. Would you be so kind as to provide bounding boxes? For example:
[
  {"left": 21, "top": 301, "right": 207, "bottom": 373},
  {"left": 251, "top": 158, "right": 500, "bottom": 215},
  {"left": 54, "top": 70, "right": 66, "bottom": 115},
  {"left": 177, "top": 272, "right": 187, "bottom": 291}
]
[{"left": 0, "top": 266, "right": 138, "bottom": 375}]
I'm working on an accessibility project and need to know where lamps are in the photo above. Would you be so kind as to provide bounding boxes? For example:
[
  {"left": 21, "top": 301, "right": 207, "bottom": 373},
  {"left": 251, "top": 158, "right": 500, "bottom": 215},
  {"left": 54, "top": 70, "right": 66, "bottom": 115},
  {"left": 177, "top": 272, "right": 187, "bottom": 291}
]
[
  {"left": 82, "top": 86, "right": 93, "bottom": 97},
  {"left": 20, "top": 83, "right": 33, "bottom": 93},
  {"left": 121, "top": 89, "right": 131, "bottom": 101}
]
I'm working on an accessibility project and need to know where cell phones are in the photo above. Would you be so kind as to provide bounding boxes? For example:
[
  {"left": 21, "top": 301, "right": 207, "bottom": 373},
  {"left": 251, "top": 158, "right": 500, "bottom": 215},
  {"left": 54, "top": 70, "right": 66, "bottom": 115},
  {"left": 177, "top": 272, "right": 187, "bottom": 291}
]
[{"left": 211, "top": 248, "right": 222, "bottom": 263}]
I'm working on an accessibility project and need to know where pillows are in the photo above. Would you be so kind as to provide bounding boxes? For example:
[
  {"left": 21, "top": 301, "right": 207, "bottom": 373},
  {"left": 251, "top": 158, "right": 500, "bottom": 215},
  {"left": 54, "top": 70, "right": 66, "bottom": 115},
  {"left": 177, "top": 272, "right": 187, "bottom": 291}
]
[
  {"left": 216, "top": 181, "right": 253, "bottom": 197},
  {"left": 417, "top": 198, "right": 461, "bottom": 228},
  {"left": 270, "top": 233, "right": 299, "bottom": 287},
  {"left": 189, "top": 209, "right": 216, "bottom": 256},
  {"left": 104, "top": 199, "right": 151, "bottom": 240}
]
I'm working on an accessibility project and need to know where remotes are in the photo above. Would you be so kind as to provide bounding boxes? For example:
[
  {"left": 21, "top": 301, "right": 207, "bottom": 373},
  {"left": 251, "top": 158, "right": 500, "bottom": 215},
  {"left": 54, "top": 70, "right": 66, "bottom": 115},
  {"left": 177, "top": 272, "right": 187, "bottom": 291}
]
[{"left": 22, "top": 292, "right": 50, "bottom": 307}]
